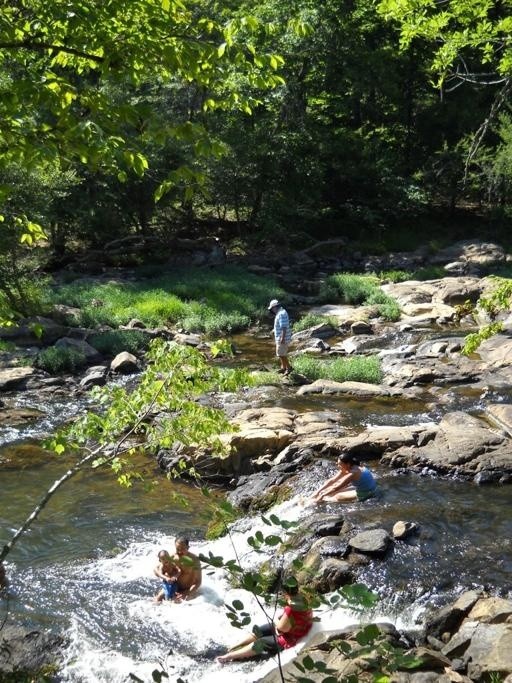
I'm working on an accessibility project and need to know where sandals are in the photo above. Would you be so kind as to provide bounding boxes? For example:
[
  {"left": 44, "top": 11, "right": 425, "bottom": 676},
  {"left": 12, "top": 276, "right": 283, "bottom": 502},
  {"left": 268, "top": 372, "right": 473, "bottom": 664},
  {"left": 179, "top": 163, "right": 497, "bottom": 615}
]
[
  {"left": 283, "top": 366, "right": 294, "bottom": 375},
  {"left": 277, "top": 368, "right": 286, "bottom": 372}
]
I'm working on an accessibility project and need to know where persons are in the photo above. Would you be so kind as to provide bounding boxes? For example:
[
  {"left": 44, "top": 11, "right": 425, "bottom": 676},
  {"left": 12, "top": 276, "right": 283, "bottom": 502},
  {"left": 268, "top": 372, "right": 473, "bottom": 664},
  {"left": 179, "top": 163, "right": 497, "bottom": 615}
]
[
  {"left": 155, "top": 535, "right": 201, "bottom": 603},
  {"left": 267, "top": 299, "right": 293, "bottom": 377},
  {"left": 299, "top": 454, "right": 378, "bottom": 506},
  {"left": 156, "top": 551, "right": 182, "bottom": 599},
  {"left": 216, "top": 576, "right": 314, "bottom": 663}
]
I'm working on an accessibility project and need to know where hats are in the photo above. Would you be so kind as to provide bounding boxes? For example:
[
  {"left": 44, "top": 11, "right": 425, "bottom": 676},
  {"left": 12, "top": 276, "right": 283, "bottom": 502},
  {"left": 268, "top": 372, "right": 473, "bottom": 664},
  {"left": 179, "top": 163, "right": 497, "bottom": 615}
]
[{"left": 268, "top": 299, "right": 280, "bottom": 309}]
